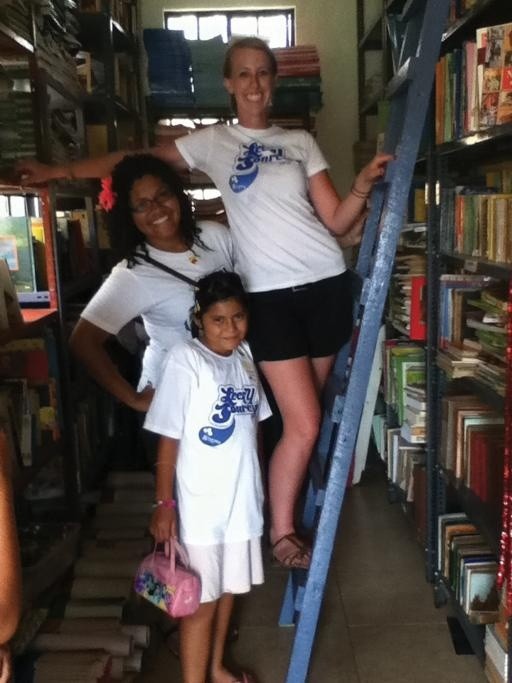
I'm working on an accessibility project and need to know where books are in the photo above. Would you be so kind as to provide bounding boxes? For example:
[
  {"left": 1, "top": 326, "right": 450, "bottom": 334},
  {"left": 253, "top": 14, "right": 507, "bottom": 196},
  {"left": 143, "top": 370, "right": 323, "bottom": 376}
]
[
  {"left": 157, "top": 27, "right": 325, "bottom": 228},
  {"left": 0, "top": 0, "right": 156, "bottom": 682},
  {"left": 354, "top": 2, "right": 510, "bottom": 681}
]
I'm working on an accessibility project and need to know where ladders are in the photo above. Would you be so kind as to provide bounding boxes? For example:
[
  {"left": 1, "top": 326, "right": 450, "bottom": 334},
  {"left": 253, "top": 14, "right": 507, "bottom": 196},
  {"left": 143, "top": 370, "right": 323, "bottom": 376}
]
[{"left": 279, "top": 0, "right": 451, "bottom": 683}]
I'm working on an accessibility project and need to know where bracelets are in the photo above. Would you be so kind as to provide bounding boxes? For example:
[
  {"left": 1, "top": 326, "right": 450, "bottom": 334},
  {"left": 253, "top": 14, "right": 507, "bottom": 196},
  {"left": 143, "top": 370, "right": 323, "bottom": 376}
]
[
  {"left": 350, "top": 183, "right": 373, "bottom": 197},
  {"left": 152, "top": 498, "right": 177, "bottom": 511}
]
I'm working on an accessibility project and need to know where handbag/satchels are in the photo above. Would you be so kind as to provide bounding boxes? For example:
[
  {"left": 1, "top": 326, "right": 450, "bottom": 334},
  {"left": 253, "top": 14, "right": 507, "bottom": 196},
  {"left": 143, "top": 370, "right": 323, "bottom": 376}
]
[{"left": 134, "top": 534, "right": 201, "bottom": 619}]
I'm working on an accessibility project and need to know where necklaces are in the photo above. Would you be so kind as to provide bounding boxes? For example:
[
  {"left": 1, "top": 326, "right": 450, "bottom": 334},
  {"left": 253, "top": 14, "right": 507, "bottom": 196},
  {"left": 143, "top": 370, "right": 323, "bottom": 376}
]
[{"left": 188, "top": 255, "right": 197, "bottom": 263}]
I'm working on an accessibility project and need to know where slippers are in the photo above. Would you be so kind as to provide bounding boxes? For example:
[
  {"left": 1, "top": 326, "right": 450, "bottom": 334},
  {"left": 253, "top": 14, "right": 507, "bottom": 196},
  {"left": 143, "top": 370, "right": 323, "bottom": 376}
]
[
  {"left": 154, "top": 620, "right": 179, "bottom": 657},
  {"left": 225, "top": 620, "right": 240, "bottom": 642}
]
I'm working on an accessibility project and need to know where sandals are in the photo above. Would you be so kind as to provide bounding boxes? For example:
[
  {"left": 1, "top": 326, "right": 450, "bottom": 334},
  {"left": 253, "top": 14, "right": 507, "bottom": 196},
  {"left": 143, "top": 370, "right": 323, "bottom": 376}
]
[{"left": 270, "top": 532, "right": 312, "bottom": 569}]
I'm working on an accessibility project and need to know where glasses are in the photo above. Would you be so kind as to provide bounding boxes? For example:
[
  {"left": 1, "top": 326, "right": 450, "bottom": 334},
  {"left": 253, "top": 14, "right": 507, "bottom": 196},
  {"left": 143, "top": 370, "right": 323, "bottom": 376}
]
[{"left": 129, "top": 185, "right": 174, "bottom": 212}]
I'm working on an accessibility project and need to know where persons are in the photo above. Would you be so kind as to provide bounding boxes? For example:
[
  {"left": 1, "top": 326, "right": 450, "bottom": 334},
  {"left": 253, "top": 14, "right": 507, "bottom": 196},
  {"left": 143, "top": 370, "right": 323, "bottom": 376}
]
[
  {"left": 74, "top": 151, "right": 372, "bottom": 663},
  {"left": 141, "top": 267, "right": 275, "bottom": 683},
  {"left": 15, "top": 32, "right": 397, "bottom": 571}
]
